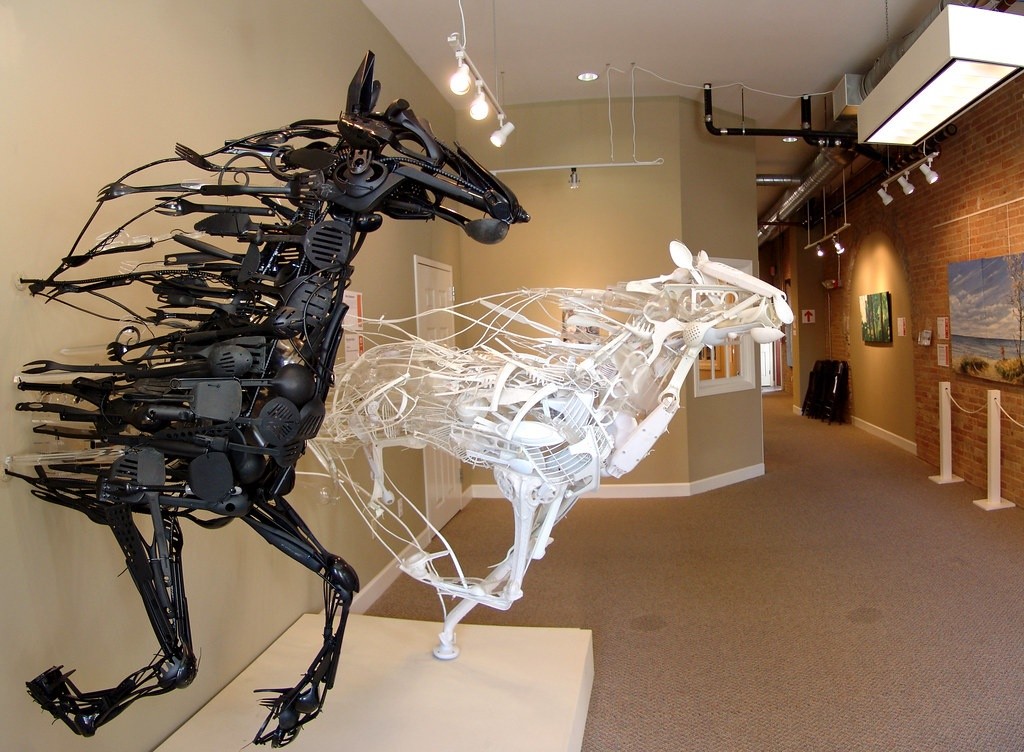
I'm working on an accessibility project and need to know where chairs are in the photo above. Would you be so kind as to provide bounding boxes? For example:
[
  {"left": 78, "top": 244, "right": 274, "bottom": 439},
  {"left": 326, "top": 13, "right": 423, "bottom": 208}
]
[{"left": 802, "top": 359, "right": 848, "bottom": 426}]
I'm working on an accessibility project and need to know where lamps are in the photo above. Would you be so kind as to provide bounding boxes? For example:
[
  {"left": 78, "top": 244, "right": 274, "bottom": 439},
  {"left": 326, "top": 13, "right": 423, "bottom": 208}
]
[
  {"left": 832, "top": 234, "right": 845, "bottom": 255},
  {"left": 919, "top": 157, "right": 939, "bottom": 185},
  {"left": 897, "top": 170, "right": 914, "bottom": 196},
  {"left": 490, "top": 114, "right": 516, "bottom": 148},
  {"left": 450, "top": 49, "right": 472, "bottom": 96},
  {"left": 469, "top": 80, "right": 489, "bottom": 121},
  {"left": 876, "top": 183, "right": 894, "bottom": 206},
  {"left": 816, "top": 242, "right": 824, "bottom": 257}
]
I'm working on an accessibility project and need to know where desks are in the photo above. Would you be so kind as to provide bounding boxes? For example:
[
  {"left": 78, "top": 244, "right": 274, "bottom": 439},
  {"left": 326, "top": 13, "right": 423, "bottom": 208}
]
[{"left": 150, "top": 613, "right": 596, "bottom": 752}]
